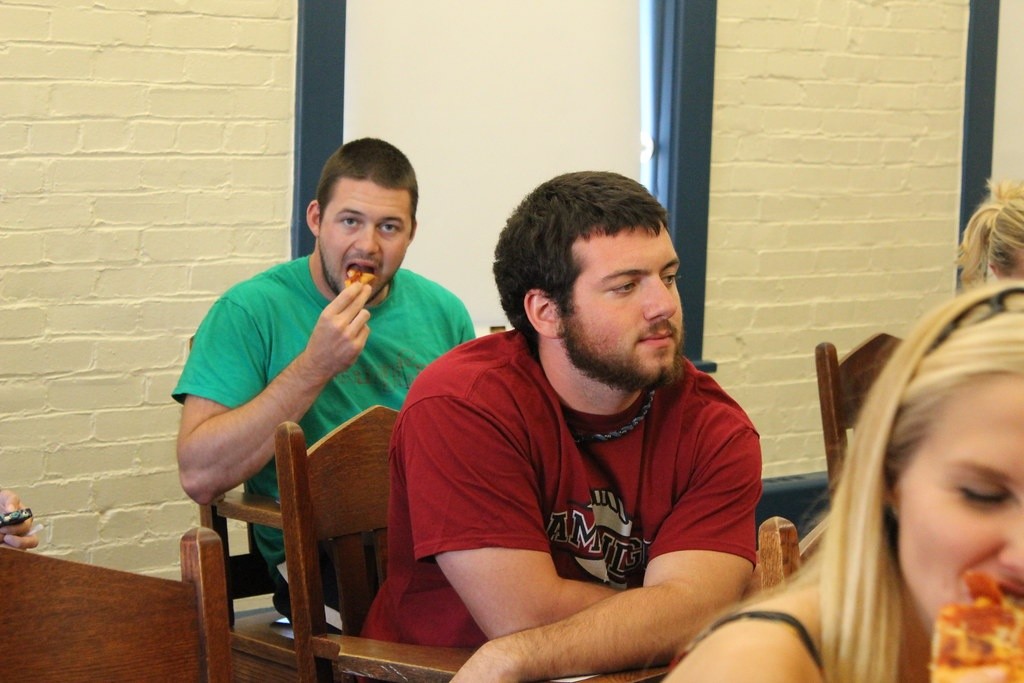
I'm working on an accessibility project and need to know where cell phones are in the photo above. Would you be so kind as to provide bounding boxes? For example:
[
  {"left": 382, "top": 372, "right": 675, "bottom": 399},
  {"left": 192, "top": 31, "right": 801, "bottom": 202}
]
[{"left": 0, "top": 508, "right": 32, "bottom": 528}]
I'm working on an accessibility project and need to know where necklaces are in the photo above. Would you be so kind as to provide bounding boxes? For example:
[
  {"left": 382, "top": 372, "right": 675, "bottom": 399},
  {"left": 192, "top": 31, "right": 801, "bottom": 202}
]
[{"left": 574, "top": 388, "right": 654, "bottom": 444}]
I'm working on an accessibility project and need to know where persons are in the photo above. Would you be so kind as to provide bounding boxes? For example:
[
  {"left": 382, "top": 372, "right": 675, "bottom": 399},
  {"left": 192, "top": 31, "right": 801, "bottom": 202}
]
[
  {"left": 171, "top": 138, "right": 472, "bottom": 627},
  {"left": 367, "top": 173, "right": 760, "bottom": 683},
  {"left": 661, "top": 285, "right": 1024, "bottom": 683},
  {"left": 0, "top": 490, "right": 40, "bottom": 553},
  {"left": 953, "top": 182, "right": 1024, "bottom": 288}
]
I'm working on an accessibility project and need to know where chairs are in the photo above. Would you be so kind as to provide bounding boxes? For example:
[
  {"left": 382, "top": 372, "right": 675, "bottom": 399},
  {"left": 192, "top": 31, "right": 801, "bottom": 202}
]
[
  {"left": 180, "top": 336, "right": 296, "bottom": 683},
  {"left": 274, "top": 405, "right": 474, "bottom": 683},
  {"left": 814, "top": 332, "right": 902, "bottom": 509},
  {"left": 0, "top": 527, "right": 234, "bottom": 683}
]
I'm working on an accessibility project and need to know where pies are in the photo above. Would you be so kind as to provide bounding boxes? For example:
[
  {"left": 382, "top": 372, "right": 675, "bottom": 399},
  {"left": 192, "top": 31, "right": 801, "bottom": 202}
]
[
  {"left": 345, "top": 270, "right": 374, "bottom": 287},
  {"left": 928, "top": 573, "right": 1024, "bottom": 682}
]
